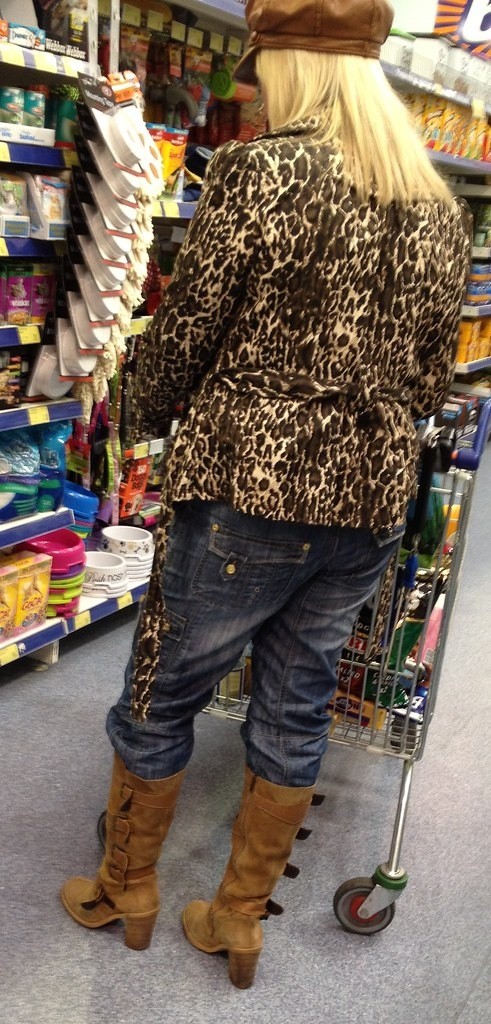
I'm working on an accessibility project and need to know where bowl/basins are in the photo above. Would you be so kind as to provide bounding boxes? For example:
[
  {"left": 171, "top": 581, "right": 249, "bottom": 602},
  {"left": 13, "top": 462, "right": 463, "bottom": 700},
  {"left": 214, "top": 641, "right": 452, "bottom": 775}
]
[{"left": 0, "top": 478, "right": 155, "bottom": 620}]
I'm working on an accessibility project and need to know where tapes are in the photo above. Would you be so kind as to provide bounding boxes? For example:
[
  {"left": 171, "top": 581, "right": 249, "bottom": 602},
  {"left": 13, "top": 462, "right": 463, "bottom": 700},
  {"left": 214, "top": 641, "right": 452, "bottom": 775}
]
[{"left": 40, "top": 116, "right": 163, "bottom": 400}]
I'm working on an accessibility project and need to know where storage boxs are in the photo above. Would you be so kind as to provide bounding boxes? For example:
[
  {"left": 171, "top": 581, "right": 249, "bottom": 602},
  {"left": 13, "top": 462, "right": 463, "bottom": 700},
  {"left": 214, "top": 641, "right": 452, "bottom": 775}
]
[
  {"left": 9, "top": 24, "right": 45, "bottom": 51},
  {"left": 454, "top": 318, "right": 491, "bottom": 362},
  {"left": 118, "top": 456, "right": 152, "bottom": 516},
  {"left": 0, "top": 171, "right": 71, "bottom": 241},
  {"left": 0, "top": 551, "right": 52, "bottom": 640},
  {"left": 0, "top": 121, "right": 55, "bottom": 147}
]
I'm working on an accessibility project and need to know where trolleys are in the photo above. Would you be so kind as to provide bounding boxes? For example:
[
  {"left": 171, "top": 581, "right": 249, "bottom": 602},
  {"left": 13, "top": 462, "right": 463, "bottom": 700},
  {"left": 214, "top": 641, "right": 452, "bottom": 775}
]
[{"left": 197, "top": 386, "right": 490, "bottom": 934}]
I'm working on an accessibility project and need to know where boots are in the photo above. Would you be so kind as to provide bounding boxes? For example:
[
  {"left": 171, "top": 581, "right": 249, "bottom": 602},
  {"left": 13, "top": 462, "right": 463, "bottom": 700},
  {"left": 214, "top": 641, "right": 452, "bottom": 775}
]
[
  {"left": 62, "top": 751, "right": 186, "bottom": 950},
  {"left": 182, "top": 763, "right": 317, "bottom": 988}
]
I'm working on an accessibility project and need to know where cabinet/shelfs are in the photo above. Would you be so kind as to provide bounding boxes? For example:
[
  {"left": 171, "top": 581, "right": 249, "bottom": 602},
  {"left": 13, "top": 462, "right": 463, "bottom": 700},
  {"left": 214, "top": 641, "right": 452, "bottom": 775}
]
[{"left": 0, "top": 1, "right": 491, "bottom": 668}]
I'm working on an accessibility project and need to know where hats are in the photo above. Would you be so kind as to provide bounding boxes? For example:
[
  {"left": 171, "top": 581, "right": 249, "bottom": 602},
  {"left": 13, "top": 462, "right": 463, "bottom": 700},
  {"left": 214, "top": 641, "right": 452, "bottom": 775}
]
[{"left": 230, "top": 0, "right": 394, "bottom": 87}]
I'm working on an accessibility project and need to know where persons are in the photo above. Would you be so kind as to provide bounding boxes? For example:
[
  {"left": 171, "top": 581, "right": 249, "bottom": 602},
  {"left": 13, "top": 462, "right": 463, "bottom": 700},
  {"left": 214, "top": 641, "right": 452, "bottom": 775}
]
[{"left": 60, "top": 0, "right": 472, "bottom": 989}]
